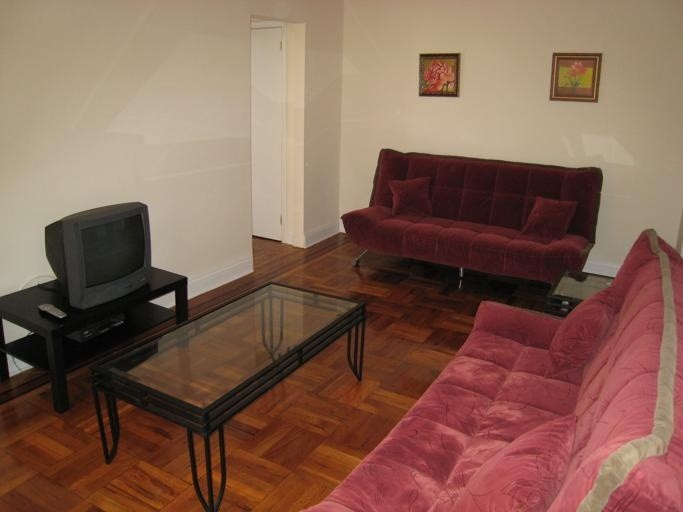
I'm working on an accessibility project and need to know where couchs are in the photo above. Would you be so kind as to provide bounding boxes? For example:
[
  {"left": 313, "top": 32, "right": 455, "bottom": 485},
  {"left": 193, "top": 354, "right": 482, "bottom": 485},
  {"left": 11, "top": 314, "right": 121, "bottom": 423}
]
[
  {"left": 296, "top": 228, "right": 683, "bottom": 511},
  {"left": 341, "top": 148, "right": 602, "bottom": 289}
]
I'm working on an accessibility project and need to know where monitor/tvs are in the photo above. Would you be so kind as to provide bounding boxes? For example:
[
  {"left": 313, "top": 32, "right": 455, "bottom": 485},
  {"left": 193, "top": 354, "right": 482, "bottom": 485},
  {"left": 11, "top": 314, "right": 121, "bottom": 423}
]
[{"left": 45, "top": 201, "right": 151, "bottom": 309}]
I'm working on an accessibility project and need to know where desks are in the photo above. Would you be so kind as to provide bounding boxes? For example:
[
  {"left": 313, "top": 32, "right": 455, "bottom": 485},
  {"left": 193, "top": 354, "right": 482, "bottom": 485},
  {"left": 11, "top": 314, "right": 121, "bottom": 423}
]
[{"left": 0, "top": 267, "right": 187, "bottom": 414}]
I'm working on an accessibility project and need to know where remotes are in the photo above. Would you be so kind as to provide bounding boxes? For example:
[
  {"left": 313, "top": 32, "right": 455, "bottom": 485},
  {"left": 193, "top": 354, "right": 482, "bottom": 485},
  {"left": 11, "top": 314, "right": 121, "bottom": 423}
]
[{"left": 38, "top": 303, "right": 67, "bottom": 320}]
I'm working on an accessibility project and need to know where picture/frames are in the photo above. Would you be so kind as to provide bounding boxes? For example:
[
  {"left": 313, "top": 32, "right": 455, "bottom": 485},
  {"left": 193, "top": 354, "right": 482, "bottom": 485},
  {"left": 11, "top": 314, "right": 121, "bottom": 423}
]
[
  {"left": 419, "top": 53, "right": 460, "bottom": 96},
  {"left": 549, "top": 52, "right": 602, "bottom": 103}
]
